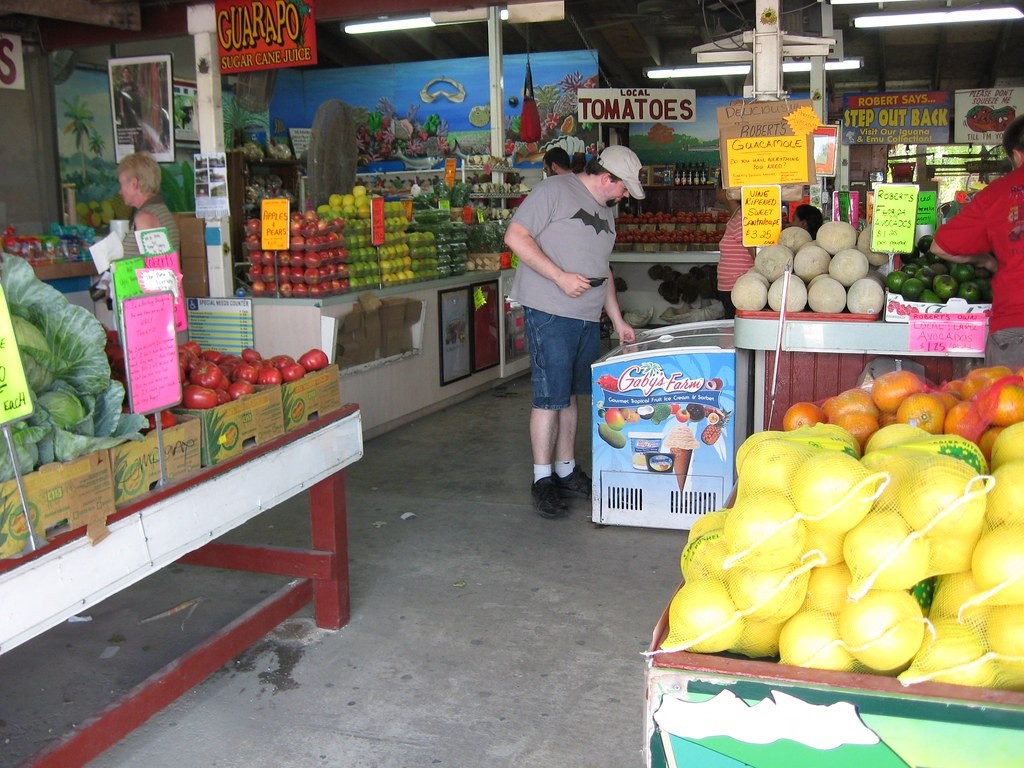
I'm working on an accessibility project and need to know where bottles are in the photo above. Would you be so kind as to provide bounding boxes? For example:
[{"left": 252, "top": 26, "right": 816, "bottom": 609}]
[
  {"left": 640, "top": 174, "right": 646, "bottom": 184},
  {"left": 504, "top": 295, "right": 530, "bottom": 359},
  {"left": 437, "top": 181, "right": 451, "bottom": 211},
  {"left": 687, "top": 164, "right": 693, "bottom": 185},
  {"left": 681, "top": 163, "right": 687, "bottom": 186},
  {"left": 711, "top": 163, "right": 716, "bottom": 177},
  {"left": 700, "top": 163, "right": 707, "bottom": 185},
  {"left": 715, "top": 163, "right": 721, "bottom": 178},
  {"left": 655, "top": 164, "right": 673, "bottom": 184},
  {"left": 694, "top": 163, "right": 699, "bottom": 185},
  {"left": 674, "top": 163, "right": 680, "bottom": 185}
]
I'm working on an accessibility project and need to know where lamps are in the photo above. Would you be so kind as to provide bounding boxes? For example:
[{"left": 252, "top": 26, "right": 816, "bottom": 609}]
[
  {"left": 341, "top": 8, "right": 509, "bottom": 35},
  {"left": 850, "top": 6, "right": 1024, "bottom": 28},
  {"left": 643, "top": 56, "right": 866, "bottom": 79}
]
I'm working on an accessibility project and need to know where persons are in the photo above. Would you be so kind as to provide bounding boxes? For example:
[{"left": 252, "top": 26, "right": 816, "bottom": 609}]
[
  {"left": 789, "top": 205, "right": 822, "bottom": 239},
  {"left": 504, "top": 144, "right": 647, "bottom": 519},
  {"left": 714, "top": 171, "right": 757, "bottom": 317},
  {"left": 99, "top": 154, "right": 183, "bottom": 331},
  {"left": 928, "top": 115, "right": 1024, "bottom": 372}
]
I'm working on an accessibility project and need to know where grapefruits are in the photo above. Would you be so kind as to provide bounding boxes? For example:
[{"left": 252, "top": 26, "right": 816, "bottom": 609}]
[{"left": 669, "top": 422, "right": 1024, "bottom": 694}]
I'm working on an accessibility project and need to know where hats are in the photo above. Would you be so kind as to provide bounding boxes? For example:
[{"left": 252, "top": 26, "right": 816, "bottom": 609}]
[{"left": 596, "top": 145, "right": 646, "bottom": 199}]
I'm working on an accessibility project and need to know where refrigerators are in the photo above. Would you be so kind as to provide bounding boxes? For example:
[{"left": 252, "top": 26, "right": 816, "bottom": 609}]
[{"left": 590, "top": 319, "right": 754, "bottom": 530}]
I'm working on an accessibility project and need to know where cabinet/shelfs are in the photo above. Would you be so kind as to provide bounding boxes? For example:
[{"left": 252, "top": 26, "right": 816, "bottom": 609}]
[
  {"left": 225, "top": 150, "right": 305, "bottom": 263},
  {"left": 300, "top": 165, "right": 522, "bottom": 224}
]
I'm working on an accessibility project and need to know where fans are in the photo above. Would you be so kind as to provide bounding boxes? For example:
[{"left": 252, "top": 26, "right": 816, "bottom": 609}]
[{"left": 307, "top": 99, "right": 358, "bottom": 211}]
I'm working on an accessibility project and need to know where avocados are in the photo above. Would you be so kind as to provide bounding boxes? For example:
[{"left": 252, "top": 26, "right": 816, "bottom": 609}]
[{"left": 886, "top": 235, "right": 996, "bottom": 305}]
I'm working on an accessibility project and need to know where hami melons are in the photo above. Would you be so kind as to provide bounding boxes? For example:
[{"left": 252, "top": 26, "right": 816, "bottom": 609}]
[{"left": 729, "top": 222, "right": 889, "bottom": 314}]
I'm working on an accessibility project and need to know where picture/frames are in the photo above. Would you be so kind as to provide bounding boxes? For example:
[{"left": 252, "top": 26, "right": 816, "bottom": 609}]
[
  {"left": 639, "top": 166, "right": 650, "bottom": 186},
  {"left": 438, "top": 286, "right": 472, "bottom": 387},
  {"left": 650, "top": 165, "right": 676, "bottom": 186},
  {"left": 470, "top": 279, "right": 500, "bottom": 374}
]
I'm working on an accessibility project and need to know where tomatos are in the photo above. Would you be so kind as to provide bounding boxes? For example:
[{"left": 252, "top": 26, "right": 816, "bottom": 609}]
[
  {"left": 98, "top": 324, "right": 329, "bottom": 434},
  {"left": 613, "top": 211, "right": 733, "bottom": 244}
]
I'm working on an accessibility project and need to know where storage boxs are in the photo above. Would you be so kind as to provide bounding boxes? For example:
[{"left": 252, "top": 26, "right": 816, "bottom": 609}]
[
  {"left": 181, "top": 257, "right": 209, "bottom": 299},
  {"left": 170, "top": 384, "right": 284, "bottom": 467},
  {"left": 108, "top": 413, "right": 202, "bottom": 504},
  {"left": 889, "top": 162, "right": 917, "bottom": 183},
  {"left": 280, "top": 364, "right": 340, "bottom": 432},
  {"left": 179, "top": 217, "right": 206, "bottom": 258},
  {"left": 0, "top": 449, "right": 118, "bottom": 560},
  {"left": 885, "top": 289, "right": 992, "bottom": 326}
]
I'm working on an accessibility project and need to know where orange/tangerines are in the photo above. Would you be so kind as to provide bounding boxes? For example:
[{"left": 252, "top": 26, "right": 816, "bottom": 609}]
[{"left": 782, "top": 365, "right": 1024, "bottom": 470}]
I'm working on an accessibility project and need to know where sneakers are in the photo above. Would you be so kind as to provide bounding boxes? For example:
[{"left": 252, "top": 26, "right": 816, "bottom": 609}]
[
  {"left": 531, "top": 472, "right": 568, "bottom": 519},
  {"left": 554, "top": 464, "right": 592, "bottom": 500}
]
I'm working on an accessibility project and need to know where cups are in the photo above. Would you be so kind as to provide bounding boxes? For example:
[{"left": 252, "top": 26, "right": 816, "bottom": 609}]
[
  {"left": 627, "top": 432, "right": 665, "bottom": 470},
  {"left": 643, "top": 453, "right": 675, "bottom": 473}
]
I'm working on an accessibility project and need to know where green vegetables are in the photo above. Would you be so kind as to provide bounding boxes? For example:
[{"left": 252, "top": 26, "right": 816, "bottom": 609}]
[{"left": 410, "top": 180, "right": 510, "bottom": 274}]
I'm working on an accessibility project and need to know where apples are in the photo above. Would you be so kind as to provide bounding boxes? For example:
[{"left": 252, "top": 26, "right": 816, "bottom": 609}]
[
  {"left": 317, "top": 187, "right": 440, "bottom": 290},
  {"left": 247, "top": 210, "right": 349, "bottom": 298}
]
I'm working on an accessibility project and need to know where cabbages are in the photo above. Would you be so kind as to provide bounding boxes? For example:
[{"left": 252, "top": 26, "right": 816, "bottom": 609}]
[{"left": 0, "top": 252, "right": 151, "bottom": 484}]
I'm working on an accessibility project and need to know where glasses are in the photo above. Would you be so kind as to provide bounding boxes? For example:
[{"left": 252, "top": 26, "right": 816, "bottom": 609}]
[
  {"left": 543, "top": 167, "right": 548, "bottom": 172},
  {"left": 586, "top": 278, "right": 608, "bottom": 287}
]
